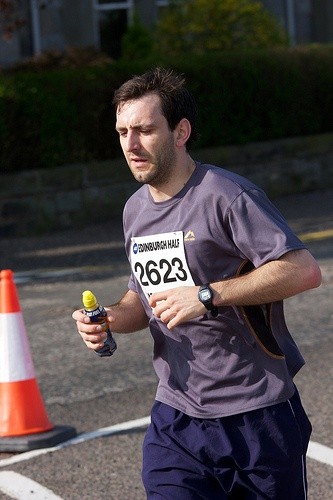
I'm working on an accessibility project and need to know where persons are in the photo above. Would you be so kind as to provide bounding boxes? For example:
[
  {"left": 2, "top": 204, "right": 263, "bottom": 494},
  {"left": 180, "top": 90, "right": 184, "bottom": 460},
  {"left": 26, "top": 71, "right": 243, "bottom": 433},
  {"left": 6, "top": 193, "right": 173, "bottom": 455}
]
[{"left": 71, "top": 66, "right": 323, "bottom": 500}]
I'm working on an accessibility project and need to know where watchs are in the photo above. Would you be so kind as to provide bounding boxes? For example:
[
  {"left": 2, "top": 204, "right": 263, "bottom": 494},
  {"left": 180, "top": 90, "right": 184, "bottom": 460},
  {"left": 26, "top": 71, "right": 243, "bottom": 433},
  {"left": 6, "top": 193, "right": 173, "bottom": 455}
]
[{"left": 198, "top": 282, "right": 219, "bottom": 317}]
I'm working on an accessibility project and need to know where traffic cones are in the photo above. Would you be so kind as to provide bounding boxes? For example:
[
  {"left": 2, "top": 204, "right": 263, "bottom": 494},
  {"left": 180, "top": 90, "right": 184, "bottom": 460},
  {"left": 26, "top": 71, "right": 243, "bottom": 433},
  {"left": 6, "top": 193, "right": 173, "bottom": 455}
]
[{"left": 0, "top": 269, "right": 78, "bottom": 452}]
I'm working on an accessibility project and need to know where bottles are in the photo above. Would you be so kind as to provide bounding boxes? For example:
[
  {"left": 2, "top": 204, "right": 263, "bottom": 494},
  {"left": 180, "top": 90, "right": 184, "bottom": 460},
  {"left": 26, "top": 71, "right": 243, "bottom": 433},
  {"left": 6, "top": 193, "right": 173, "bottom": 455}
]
[{"left": 82, "top": 290, "right": 117, "bottom": 357}]
